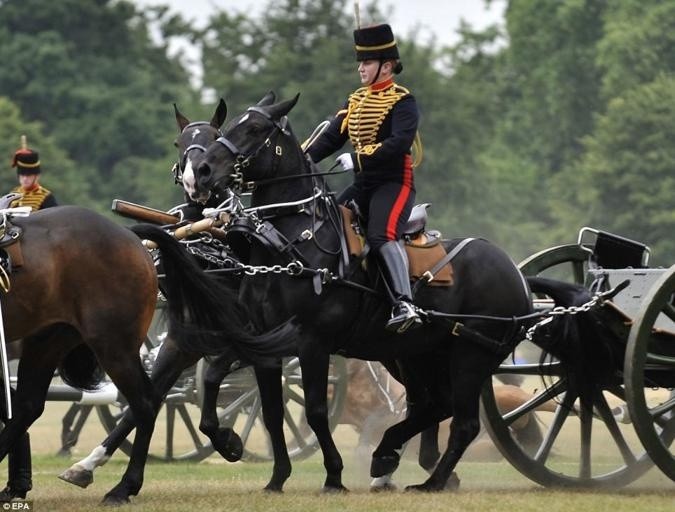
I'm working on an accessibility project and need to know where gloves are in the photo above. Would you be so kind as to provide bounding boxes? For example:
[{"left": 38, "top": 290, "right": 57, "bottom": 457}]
[{"left": 335, "top": 153, "right": 354, "bottom": 171}]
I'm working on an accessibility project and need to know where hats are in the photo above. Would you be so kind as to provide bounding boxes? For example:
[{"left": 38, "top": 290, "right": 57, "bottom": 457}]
[
  {"left": 354, "top": 23, "right": 400, "bottom": 61},
  {"left": 12, "top": 149, "right": 41, "bottom": 174}
]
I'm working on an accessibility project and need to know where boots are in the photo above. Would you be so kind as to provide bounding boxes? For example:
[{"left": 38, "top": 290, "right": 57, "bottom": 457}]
[{"left": 374, "top": 299, "right": 423, "bottom": 329}]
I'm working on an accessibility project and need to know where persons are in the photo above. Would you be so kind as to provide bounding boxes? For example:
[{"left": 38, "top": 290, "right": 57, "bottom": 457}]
[
  {"left": 300, "top": 24, "right": 423, "bottom": 333},
  {"left": 9, "top": 149, "right": 58, "bottom": 212}
]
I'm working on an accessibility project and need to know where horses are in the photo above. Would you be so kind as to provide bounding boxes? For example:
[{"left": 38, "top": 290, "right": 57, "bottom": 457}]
[{"left": 0, "top": 90, "right": 632, "bottom": 507}]
